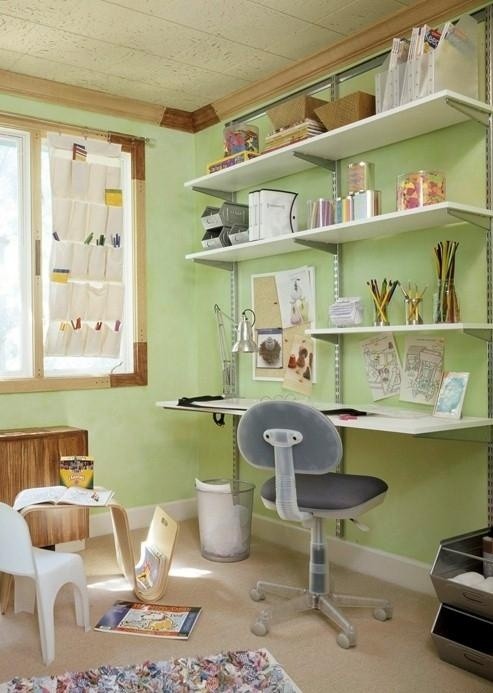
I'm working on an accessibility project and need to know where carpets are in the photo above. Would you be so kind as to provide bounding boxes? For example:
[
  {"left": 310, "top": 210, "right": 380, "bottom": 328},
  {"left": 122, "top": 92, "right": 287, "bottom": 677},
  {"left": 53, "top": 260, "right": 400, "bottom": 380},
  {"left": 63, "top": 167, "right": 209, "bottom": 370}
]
[{"left": 0, "top": 645, "right": 304, "bottom": 692}]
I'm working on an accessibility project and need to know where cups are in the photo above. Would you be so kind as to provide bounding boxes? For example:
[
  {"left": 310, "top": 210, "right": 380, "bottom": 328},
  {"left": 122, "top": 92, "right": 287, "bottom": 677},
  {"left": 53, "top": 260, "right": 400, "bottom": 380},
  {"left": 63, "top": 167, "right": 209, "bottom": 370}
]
[
  {"left": 404, "top": 298, "right": 423, "bottom": 324},
  {"left": 394, "top": 168, "right": 446, "bottom": 210},
  {"left": 432, "top": 279, "right": 459, "bottom": 323},
  {"left": 221, "top": 123, "right": 260, "bottom": 157},
  {"left": 373, "top": 300, "right": 389, "bottom": 325}
]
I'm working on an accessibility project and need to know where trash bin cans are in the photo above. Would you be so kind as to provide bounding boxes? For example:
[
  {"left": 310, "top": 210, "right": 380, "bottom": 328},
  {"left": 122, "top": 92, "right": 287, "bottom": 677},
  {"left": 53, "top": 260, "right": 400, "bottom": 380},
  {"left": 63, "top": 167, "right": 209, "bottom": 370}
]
[{"left": 194, "top": 479, "right": 256, "bottom": 562}]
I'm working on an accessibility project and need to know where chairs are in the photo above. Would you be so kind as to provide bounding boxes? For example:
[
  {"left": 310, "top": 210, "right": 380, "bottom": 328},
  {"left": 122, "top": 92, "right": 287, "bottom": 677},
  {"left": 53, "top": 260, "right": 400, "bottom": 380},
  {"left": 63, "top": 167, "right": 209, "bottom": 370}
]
[
  {"left": 0, "top": 500, "right": 92, "bottom": 666},
  {"left": 235, "top": 398, "right": 392, "bottom": 652}
]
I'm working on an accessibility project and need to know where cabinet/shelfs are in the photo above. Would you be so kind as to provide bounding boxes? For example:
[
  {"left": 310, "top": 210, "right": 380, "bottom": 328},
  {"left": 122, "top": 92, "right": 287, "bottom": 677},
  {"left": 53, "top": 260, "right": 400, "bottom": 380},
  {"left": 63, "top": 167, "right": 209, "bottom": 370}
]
[
  {"left": 182, "top": 85, "right": 490, "bottom": 339},
  {"left": 0, "top": 423, "right": 90, "bottom": 549}
]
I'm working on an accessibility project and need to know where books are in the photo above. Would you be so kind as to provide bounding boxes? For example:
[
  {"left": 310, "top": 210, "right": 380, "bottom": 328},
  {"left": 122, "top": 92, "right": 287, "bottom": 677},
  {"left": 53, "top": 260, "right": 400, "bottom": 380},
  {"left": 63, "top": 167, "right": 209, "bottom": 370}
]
[
  {"left": 92, "top": 543, "right": 203, "bottom": 640},
  {"left": 12, "top": 485, "right": 115, "bottom": 511}
]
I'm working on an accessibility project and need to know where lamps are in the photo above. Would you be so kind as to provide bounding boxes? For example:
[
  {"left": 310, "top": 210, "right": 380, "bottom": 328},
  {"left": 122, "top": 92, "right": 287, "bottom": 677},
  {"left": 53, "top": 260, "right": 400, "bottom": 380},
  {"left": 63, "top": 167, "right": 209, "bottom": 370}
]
[{"left": 211, "top": 301, "right": 260, "bottom": 393}]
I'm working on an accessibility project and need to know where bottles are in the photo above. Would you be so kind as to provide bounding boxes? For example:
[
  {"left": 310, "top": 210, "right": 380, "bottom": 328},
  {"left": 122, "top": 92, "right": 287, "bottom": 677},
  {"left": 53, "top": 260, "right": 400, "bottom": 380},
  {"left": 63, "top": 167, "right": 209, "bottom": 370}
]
[{"left": 287, "top": 354, "right": 296, "bottom": 367}]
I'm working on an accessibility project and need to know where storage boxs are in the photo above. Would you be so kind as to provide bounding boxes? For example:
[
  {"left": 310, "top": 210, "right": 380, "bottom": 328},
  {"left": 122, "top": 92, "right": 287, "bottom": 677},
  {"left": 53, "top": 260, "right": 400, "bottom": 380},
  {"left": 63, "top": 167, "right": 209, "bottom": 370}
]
[
  {"left": 428, "top": 601, "right": 492, "bottom": 683},
  {"left": 373, "top": 47, "right": 481, "bottom": 114},
  {"left": 426, "top": 524, "right": 491, "bottom": 620}
]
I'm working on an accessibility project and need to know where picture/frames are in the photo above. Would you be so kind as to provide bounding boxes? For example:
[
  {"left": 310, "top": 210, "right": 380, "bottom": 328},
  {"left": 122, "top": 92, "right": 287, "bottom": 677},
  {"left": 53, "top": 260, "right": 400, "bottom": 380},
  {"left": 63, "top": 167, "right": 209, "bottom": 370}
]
[{"left": 432, "top": 368, "right": 472, "bottom": 420}]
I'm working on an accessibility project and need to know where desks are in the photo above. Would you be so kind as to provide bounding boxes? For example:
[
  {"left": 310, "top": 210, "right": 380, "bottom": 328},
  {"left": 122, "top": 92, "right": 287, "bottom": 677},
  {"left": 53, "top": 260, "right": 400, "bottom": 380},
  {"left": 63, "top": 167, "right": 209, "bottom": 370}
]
[
  {"left": 2, "top": 487, "right": 183, "bottom": 615},
  {"left": 155, "top": 391, "right": 492, "bottom": 541}
]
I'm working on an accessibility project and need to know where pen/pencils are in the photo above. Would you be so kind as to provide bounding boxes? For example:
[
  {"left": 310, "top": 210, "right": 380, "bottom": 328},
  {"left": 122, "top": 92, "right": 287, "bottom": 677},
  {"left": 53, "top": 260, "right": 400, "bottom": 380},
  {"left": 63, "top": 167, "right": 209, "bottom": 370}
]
[
  {"left": 433, "top": 240, "right": 459, "bottom": 323},
  {"left": 367, "top": 279, "right": 428, "bottom": 322}
]
